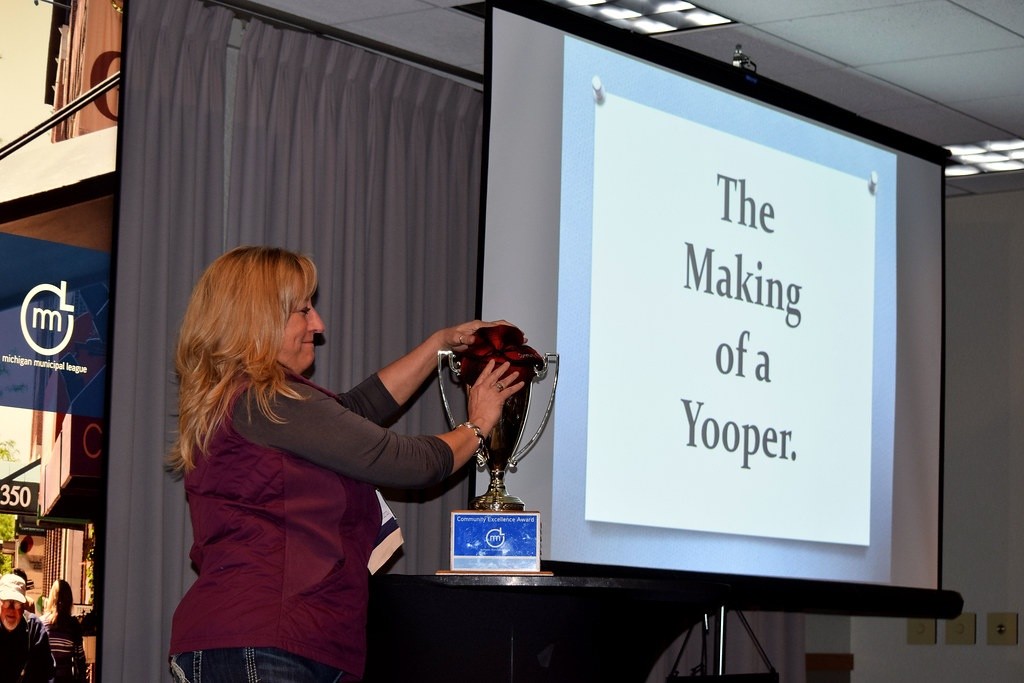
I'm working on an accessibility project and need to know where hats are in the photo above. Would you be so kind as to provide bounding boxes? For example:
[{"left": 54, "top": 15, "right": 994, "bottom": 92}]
[{"left": 0, "top": 574, "right": 27, "bottom": 603}]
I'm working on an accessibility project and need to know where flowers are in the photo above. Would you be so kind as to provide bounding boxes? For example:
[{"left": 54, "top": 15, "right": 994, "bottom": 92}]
[{"left": 460, "top": 324, "right": 543, "bottom": 383}]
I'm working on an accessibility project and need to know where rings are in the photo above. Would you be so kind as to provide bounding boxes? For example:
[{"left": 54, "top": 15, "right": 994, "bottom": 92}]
[
  {"left": 494, "top": 382, "right": 503, "bottom": 391},
  {"left": 458, "top": 335, "right": 465, "bottom": 345}
]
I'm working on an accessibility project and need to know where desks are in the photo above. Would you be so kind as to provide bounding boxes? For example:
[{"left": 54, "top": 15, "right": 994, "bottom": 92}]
[{"left": 365, "top": 575, "right": 653, "bottom": 682}]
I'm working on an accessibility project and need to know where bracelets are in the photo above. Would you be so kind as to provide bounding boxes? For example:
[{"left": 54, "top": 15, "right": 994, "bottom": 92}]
[{"left": 455, "top": 421, "right": 485, "bottom": 456}]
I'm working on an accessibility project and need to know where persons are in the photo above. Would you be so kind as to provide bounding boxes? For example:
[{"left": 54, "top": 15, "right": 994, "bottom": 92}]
[
  {"left": 40, "top": 580, "right": 87, "bottom": 683},
  {"left": 11, "top": 569, "right": 36, "bottom": 613},
  {"left": 167, "top": 244, "right": 529, "bottom": 683},
  {"left": 0, "top": 573, "right": 56, "bottom": 682}
]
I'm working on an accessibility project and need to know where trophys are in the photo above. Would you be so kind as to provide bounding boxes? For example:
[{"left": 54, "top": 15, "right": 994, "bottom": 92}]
[{"left": 435, "top": 326, "right": 559, "bottom": 578}]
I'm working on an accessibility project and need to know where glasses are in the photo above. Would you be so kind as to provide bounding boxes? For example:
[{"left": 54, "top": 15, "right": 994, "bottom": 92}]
[{"left": 1, "top": 601, "right": 22, "bottom": 609}]
